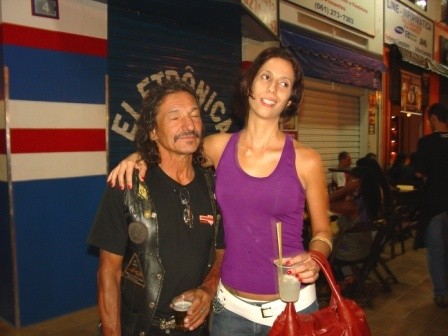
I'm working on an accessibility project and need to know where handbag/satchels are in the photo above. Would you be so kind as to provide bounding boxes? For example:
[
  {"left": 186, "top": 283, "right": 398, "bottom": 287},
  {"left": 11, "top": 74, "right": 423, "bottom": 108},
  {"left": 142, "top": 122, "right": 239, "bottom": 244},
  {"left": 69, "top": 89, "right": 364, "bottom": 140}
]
[{"left": 266, "top": 250, "right": 373, "bottom": 336}]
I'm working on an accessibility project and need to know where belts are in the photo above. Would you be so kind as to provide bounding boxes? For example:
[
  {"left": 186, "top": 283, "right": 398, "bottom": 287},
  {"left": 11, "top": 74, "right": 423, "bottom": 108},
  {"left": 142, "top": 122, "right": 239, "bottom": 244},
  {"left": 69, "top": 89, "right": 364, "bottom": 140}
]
[{"left": 216, "top": 276, "right": 317, "bottom": 328}]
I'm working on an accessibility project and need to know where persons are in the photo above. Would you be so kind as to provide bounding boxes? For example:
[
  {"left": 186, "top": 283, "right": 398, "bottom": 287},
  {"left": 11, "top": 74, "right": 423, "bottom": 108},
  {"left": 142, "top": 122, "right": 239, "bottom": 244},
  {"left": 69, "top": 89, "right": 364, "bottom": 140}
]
[
  {"left": 413, "top": 102, "right": 448, "bottom": 313},
  {"left": 109, "top": 46, "right": 334, "bottom": 336},
  {"left": 88, "top": 80, "right": 225, "bottom": 336},
  {"left": 304, "top": 151, "right": 426, "bottom": 301}
]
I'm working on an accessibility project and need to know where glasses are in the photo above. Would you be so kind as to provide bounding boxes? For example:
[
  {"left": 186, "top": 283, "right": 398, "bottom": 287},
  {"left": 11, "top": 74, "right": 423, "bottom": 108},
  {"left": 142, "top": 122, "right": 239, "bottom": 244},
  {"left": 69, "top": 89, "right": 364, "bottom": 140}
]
[{"left": 179, "top": 186, "right": 194, "bottom": 228}]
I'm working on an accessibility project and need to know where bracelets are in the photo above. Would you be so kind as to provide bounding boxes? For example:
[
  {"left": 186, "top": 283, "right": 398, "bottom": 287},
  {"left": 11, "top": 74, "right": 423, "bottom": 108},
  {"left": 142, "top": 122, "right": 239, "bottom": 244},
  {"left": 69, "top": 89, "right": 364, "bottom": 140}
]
[{"left": 309, "top": 237, "right": 334, "bottom": 253}]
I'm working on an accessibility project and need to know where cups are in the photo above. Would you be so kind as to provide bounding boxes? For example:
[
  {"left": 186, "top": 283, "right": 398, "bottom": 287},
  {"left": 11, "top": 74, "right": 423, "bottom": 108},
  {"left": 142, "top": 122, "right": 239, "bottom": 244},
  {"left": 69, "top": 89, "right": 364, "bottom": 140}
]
[
  {"left": 173, "top": 301, "right": 192, "bottom": 332},
  {"left": 273, "top": 259, "right": 302, "bottom": 303}
]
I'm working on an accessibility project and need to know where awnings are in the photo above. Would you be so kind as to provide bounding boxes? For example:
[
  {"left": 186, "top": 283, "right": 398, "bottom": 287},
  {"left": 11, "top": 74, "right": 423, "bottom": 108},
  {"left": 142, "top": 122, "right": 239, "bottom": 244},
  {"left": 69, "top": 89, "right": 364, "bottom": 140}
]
[
  {"left": 94, "top": 0, "right": 277, "bottom": 43},
  {"left": 279, "top": 28, "right": 383, "bottom": 91},
  {"left": 392, "top": 45, "right": 448, "bottom": 79}
]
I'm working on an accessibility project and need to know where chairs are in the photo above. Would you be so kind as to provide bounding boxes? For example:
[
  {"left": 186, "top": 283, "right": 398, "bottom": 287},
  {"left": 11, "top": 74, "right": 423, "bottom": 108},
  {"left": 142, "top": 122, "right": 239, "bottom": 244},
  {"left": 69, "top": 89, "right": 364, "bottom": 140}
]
[{"left": 328, "top": 187, "right": 429, "bottom": 295}]
